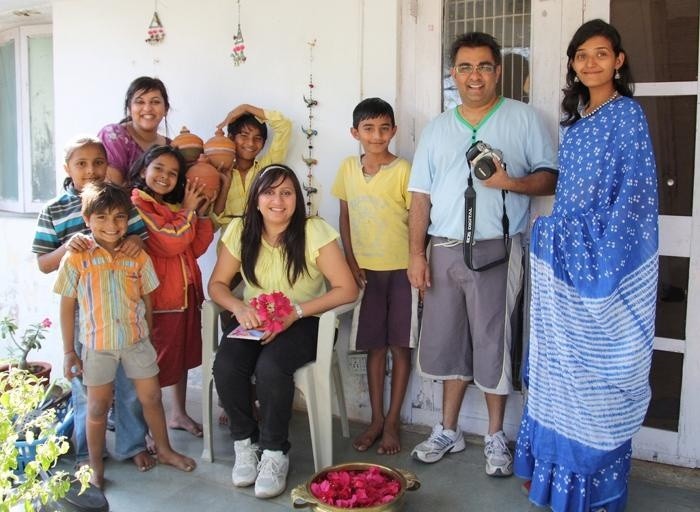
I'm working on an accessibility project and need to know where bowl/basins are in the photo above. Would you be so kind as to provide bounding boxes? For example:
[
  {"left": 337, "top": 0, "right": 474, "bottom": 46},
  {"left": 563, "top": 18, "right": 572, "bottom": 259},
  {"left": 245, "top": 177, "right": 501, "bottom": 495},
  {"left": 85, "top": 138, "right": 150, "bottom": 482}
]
[{"left": 291, "top": 464, "right": 423, "bottom": 511}]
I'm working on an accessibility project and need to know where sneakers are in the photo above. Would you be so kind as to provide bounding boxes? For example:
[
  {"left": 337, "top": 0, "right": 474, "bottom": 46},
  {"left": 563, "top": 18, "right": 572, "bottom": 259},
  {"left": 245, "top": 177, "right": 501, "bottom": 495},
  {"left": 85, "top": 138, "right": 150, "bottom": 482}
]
[
  {"left": 255, "top": 450, "right": 291, "bottom": 500},
  {"left": 485, "top": 433, "right": 515, "bottom": 477},
  {"left": 410, "top": 423, "right": 465, "bottom": 464},
  {"left": 230, "top": 440, "right": 260, "bottom": 488},
  {"left": 104, "top": 408, "right": 117, "bottom": 432}
]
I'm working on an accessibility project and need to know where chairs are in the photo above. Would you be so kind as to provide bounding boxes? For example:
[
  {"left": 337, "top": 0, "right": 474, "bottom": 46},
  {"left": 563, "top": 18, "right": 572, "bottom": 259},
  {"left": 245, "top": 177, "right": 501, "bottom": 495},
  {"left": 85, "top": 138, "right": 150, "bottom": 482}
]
[{"left": 201, "top": 277, "right": 364, "bottom": 472}]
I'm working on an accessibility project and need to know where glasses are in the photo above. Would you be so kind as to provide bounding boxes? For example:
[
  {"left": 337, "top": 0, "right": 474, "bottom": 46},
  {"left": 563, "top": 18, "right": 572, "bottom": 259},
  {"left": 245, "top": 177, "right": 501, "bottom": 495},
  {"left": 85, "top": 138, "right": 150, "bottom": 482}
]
[{"left": 452, "top": 65, "right": 494, "bottom": 75}]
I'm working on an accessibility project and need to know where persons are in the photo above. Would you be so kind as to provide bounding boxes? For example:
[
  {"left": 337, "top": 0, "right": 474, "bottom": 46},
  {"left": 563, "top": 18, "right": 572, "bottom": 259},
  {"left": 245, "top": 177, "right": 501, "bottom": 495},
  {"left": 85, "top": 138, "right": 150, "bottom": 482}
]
[
  {"left": 514, "top": 19, "right": 658, "bottom": 511},
  {"left": 52, "top": 181, "right": 196, "bottom": 492},
  {"left": 408, "top": 33, "right": 558, "bottom": 477},
  {"left": 94, "top": 77, "right": 172, "bottom": 431},
  {"left": 207, "top": 165, "right": 359, "bottom": 499},
  {"left": 209, "top": 104, "right": 291, "bottom": 427},
  {"left": 332, "top": 97, "right": 419, "bottom": 455},
  {"left": 31, "top": 138, "right": 157, "bottom": 472},
  {"left": 125, "top": 144, "right": 217, "bottom": 454}
]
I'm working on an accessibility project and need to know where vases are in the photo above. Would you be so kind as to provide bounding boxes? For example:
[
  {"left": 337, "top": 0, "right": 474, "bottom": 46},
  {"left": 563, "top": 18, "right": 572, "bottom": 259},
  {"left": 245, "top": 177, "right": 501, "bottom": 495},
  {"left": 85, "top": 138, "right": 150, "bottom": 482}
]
[{"left": 0, "top": 362, "right": 52, "bottom": 388}]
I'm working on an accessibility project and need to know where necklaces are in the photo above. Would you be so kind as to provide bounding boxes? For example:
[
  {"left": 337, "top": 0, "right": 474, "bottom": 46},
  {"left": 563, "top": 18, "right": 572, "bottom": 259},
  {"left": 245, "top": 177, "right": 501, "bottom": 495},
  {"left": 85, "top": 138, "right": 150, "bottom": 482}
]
[{"left": 579, "top": 90, "right": 619, "bottom": 119}]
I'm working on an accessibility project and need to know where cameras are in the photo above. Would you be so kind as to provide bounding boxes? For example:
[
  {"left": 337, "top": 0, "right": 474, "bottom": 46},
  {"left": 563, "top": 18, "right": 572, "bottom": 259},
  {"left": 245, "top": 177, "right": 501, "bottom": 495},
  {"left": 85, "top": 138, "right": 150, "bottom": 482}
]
[{"left": 466, "top": 140, "right": 507, "bottom": 181}]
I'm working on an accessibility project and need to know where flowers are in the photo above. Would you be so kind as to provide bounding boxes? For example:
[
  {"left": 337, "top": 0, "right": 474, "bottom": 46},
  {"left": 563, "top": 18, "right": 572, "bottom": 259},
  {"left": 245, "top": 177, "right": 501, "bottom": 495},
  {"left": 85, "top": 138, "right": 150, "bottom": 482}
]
[
  {"left": 0, "top": 318, "right": 52, "bottom": 369},
  {"left": 248, "top": 290, "right": 294, "bottom": 334}
]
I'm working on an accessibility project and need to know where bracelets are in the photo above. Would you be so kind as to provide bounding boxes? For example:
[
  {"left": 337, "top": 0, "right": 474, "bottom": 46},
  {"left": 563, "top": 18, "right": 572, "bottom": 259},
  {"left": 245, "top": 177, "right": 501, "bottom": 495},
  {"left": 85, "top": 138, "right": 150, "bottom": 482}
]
[{"left": 294, "top": 304, "right": 304, "bottom": 319}]
